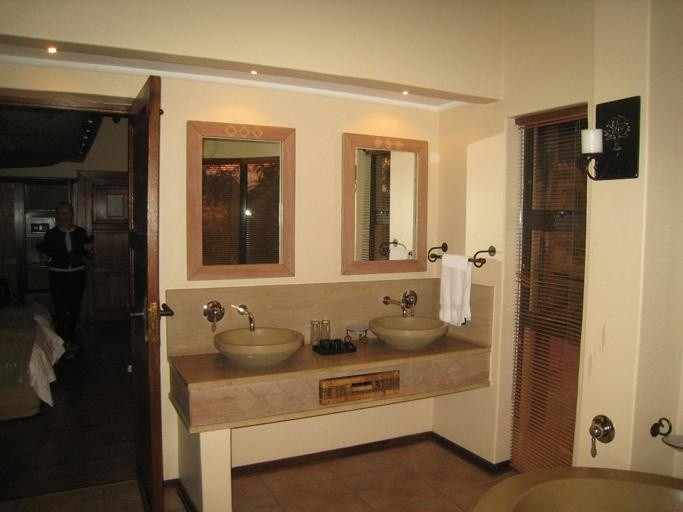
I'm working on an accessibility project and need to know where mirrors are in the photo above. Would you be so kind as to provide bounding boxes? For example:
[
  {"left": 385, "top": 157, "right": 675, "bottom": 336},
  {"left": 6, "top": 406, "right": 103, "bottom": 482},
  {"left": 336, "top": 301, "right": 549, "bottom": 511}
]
[
  {"left": 341, "top": 132, "right": 427, "bottom": 275},
  {"left": 187, "top": 121, "right": 295, "bottom": 281}
]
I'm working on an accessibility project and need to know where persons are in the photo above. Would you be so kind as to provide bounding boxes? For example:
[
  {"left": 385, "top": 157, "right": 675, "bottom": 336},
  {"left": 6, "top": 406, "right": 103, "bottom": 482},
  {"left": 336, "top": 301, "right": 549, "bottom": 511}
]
[{"left": 38, "top": 201, "right": 96, "bottom": 363}]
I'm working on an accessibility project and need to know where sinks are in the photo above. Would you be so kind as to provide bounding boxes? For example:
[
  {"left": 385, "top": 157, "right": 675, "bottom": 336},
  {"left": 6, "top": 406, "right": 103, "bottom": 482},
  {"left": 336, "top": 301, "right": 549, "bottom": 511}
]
[
  {"left": 212, "top": 327, "right": 304, "bottom": 367},
  {"left": 369, "top": 316, "right": 449, "bottom": 350}
]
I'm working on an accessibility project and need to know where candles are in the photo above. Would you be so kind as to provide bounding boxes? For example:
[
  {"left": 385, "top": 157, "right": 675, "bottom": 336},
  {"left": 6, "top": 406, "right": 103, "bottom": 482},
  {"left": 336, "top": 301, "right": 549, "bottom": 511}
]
[{"left": 581, "top": 129, "right": 603, "bottom": 153}]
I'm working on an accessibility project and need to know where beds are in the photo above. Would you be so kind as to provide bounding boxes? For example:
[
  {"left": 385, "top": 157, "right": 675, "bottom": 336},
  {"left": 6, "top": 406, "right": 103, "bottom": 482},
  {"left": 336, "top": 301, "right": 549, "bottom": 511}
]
[{"left": 0, "top": 304, "right": 66, "bottom": 422}]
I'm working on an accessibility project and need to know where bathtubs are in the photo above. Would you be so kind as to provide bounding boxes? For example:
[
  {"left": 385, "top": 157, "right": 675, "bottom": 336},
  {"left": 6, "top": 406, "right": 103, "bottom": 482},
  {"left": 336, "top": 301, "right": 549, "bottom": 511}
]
[{"left": 470, "top": 466, "right": 683, "bottom": 512}]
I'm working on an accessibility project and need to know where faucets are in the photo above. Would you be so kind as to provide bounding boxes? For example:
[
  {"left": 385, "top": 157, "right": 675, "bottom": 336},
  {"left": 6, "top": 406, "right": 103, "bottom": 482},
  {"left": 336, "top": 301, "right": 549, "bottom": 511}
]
[
  {"left": 382, "top": 296, "right": 407, "bottom": 319},
  {"left": 230, "top": 304, "right": 256, "bottom": 332}
]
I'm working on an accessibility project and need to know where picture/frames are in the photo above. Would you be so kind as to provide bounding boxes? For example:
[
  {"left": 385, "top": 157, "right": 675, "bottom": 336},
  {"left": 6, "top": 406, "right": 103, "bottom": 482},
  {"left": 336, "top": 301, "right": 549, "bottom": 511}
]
[{"left": 106, "top": 193, "right": 124, "bottom": 217}]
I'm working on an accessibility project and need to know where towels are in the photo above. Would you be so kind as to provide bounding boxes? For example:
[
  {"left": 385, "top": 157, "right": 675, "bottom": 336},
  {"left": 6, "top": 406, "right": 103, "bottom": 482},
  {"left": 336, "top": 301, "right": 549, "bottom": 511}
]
[
  {"left": 389, "top": 248, "right": 411, "bottom": 260},
  {"left": 439, "top": 254, "right": 473, "bottom": 327}
]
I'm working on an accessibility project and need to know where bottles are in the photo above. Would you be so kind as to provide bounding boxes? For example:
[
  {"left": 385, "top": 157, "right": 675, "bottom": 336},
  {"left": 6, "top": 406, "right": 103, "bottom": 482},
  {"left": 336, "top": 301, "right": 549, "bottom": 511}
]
[{"left": 311, "top": 320, "right": 329, "bottom": 347}]
